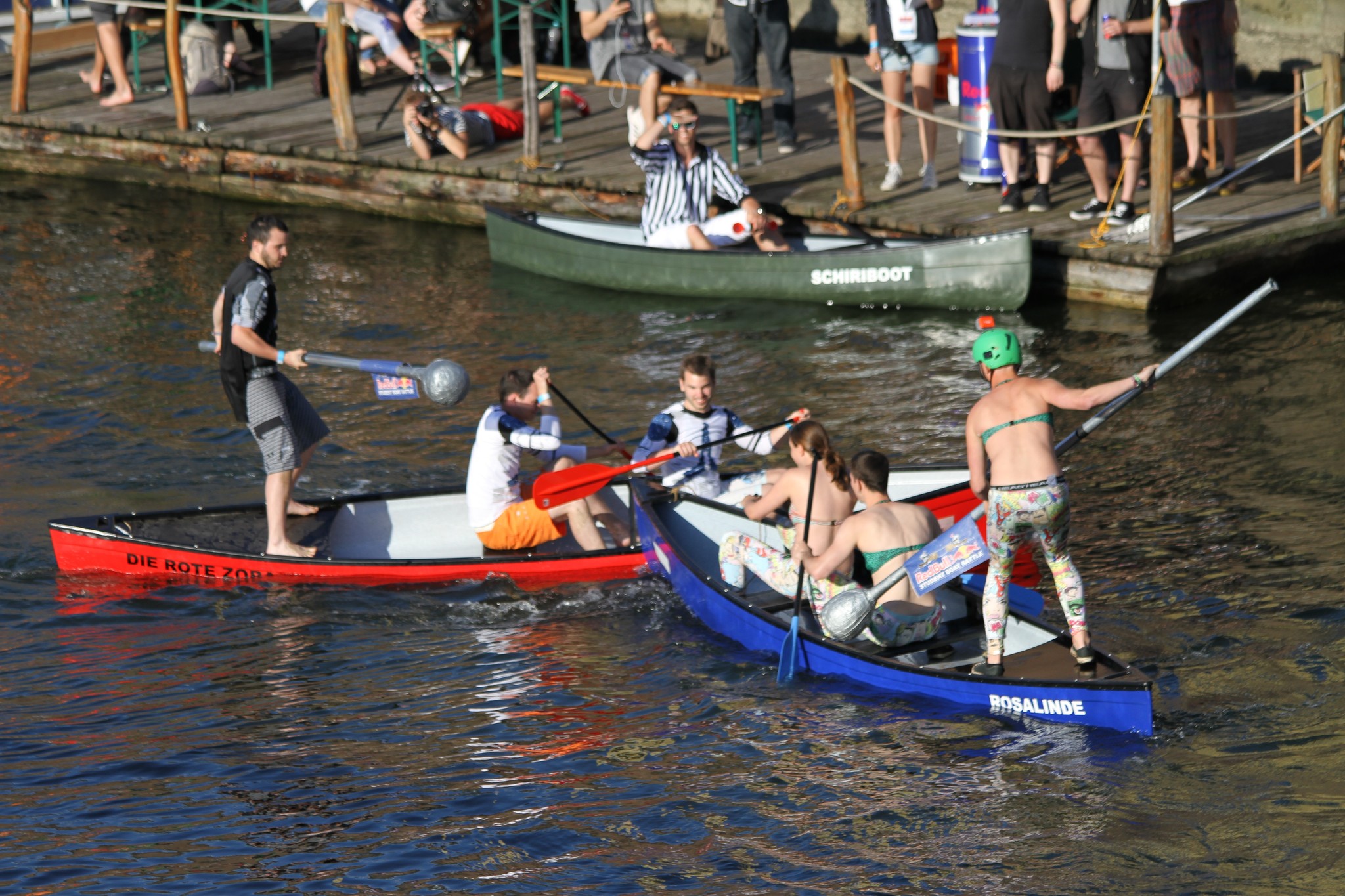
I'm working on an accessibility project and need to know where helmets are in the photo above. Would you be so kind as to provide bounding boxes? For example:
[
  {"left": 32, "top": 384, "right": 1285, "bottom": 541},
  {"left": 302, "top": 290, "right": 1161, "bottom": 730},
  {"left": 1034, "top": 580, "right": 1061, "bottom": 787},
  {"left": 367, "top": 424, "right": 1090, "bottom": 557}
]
[{"left": 972, "top": 329, "right": 1022, "bottom": 370}]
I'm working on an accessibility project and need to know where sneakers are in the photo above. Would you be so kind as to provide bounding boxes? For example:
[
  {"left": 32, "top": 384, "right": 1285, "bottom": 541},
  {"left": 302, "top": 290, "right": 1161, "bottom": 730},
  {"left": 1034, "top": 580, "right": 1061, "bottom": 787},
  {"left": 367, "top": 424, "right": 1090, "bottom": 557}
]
[
  {"left": 1107, "top": 202, "right": 1135, "bottom": 225},
  {"left": 1069, "top": 197, "right": 1114, "bottom": 221}
]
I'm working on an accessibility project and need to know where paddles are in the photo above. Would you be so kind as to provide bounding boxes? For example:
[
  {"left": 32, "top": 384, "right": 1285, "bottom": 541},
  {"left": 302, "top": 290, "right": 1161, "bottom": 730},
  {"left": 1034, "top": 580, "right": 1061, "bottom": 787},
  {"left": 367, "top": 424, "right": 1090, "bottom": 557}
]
[
  {"left": 531, "top": 409, "right": 808, "bottom": 510},
  {"left": 775, "top": 455, "right": 821, "bottom": 685},
  {"left": 546, "top": 383, "right": 634, "bottom": 463},
  {"left": 753, "top": 494, "right": 790, "bottom": 519}
]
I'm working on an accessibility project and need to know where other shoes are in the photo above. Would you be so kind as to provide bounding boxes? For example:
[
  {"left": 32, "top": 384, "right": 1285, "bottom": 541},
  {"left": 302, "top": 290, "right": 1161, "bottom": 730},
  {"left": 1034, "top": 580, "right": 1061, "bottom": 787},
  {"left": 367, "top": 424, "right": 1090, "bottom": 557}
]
[
  {"left": 1171, "top": 167, "right": 1206, "bottom": 192},
  {"left": 1070, "top": 642, "right": 1095, "bottom": 664},
  {"left": 927, "top": 645, "right": 955, "bottom": 659},
  {"left": 879, "top": 161, "right": 903, "bottom": 191},
  {"left": 1217, "top": 167, "right": 1240, "bottom": 196},
  {"left": 1028, "top": 188, "right": 1051, "bottom": 212},
  {"left": 560, "top": 85, "right": 591, "bottom": 119},
  {"left": 737, "top": 136, "right": 757, "bottom": 151},
  {"left": 972, "top": 656, "right": 1005, "bottom": 676},
  {"left": 777, "top": 141, "right": 797, "bottom": 153},
  {"left": 998, "top": 189, "right": 1024, "bottom": 212},
  {"left": 918, "top": 163, "right": 940, "bottom": 189}
]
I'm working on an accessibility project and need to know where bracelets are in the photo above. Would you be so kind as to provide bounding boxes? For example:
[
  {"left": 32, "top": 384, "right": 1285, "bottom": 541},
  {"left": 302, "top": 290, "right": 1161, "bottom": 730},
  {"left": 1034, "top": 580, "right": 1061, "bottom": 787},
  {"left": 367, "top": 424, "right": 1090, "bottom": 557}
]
[
  {"left": 434, "top": 124, "right": 443, "bottom": 135},
  {"left": 656, "top": 110, "right": 671, "bottom": 129},
  {"left": 211, "top": 332, "right": 222, "bottom": 336},
  {"left": 870, "top": 49, "right": 879, "bottom": 54},
  {"left": 1049, "top": 60, "right": 1062, "bottom": 69},
  {"left": 1131, "top": 372, "right": 1148, "bottom": 389},
  {"left": 537, "top": 392, "right": 551, "bottom": 404},
  {"left": 277, "top": 348, "right": 286, "bottom": 365},
  {"left": 785, "top": 419, "right": 795, "bottom": 431},
  {"left": 868, "top": 40, "right": 879, "bottom": 49}
]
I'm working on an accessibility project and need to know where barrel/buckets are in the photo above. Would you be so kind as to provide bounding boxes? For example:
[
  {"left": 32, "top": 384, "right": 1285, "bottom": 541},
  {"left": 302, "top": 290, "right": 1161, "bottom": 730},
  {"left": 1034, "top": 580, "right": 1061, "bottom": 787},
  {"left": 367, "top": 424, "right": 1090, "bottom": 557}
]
[{"left": 957, "top": 13, "right": 1031, "bottom": 185}]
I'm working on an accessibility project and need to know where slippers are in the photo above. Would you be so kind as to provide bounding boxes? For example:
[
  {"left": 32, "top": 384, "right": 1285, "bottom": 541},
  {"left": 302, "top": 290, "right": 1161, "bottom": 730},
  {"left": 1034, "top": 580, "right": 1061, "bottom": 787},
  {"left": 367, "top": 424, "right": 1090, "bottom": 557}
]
[
  {"left": 1092, "top": 175, "right": 1122, "bottom": 192},
  {"left": 1136, "top": 172, "right": 1150, "bottom": 189}
]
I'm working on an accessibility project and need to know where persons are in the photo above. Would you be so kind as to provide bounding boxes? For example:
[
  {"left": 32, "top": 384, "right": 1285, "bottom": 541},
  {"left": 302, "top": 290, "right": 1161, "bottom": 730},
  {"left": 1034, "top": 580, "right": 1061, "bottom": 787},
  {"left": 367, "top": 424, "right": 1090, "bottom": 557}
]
[
  {"left": 212, "top": 214, "right": 332, "bottom": 557},
  {"left": 629, "top": 355, "right": 811, "bottom": 520},
  {"left": 718, "top": 419, "right": 858, "bottom": 601},
  {"left": 465, "top": 366, "right": 641, "bottom": 551},
  {"left": 988, "top": 0, "right": 1241, "bottom": 228},
  {"left": 790, "top": 450, "right": 956, "bottom": 661},
  {"left": 865, "top": 0, "right": 945, "bottom": 193},
  {"left": 722, "top": 0, "right": 798, "bottom": 155},
  {"left": 628, "top": 99, "right": 790, "bottom": 252},
  {"left": 574, "top": 0, "right": 700, "bottom": 149},
  {"left": 966, "top": 315, "right": 1160, "bottom": 681},
  {"left": 78, "top": 0, "right": 591, "bottom": 160}
]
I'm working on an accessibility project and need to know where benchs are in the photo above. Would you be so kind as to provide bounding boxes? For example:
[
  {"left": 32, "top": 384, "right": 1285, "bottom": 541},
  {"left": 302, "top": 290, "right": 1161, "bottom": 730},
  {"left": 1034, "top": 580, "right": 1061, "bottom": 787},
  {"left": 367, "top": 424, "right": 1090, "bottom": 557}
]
[
  {"left": 502, "top": 62, "right": 785, "bottom": 173},
  {"left": 744, "top": 544, "right": 985, "bottom": 658},
  {"left": 419, "top": 20, "right": 465, "bottom": 99}
]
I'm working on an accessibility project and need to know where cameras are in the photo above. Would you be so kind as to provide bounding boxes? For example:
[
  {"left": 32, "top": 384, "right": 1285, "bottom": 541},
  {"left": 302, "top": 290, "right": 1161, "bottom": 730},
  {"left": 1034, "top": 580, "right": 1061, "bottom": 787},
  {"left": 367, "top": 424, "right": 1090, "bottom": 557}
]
[{"left": 416, "top": 101, "right": 432, "bottom": 119}]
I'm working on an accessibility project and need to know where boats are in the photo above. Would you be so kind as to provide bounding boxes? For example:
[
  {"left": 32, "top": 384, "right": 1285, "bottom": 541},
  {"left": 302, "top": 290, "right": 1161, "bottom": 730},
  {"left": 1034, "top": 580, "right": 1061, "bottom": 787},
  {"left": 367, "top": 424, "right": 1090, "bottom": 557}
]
[
  {"left": 47, "top": 480, "right": 651, "bottom": 586},
  {"left": 482, "top": 198, "right": 1034, "bottom": 311},
  {"left": 623, "top": 474, "right": 1157, "bottom": 738}
]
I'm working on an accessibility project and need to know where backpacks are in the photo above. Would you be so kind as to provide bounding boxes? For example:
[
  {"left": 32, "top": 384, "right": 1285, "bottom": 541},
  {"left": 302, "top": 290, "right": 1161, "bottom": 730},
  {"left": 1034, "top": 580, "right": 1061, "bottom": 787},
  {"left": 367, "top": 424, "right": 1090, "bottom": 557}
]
[
  {"left": 312, "top": 35, "right": 361, "bottom": 97},
  {"left": 178, "top": 20, "right": 233, "bottom": 97}
]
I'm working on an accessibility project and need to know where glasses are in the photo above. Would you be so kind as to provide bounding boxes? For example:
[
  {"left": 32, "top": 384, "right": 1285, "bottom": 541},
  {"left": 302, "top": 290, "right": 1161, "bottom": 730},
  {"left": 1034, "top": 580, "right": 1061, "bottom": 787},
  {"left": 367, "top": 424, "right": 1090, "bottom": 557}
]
[{"left": 673, "top": 122, "right": 693, "bottom": 130}]
